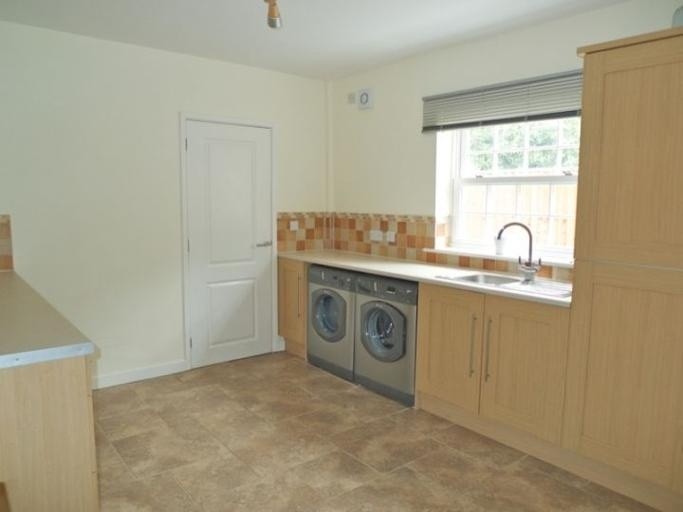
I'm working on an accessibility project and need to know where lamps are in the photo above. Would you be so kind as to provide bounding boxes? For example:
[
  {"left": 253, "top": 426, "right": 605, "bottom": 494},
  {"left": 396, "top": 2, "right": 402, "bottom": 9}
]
[{"left": 262, "top": 0, "right": 282, "bottom": 29}]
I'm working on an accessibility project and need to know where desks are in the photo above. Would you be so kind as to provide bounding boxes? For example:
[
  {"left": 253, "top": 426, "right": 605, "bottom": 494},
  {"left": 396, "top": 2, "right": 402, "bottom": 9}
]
[{"left": 0, "top": 214, "right": 102, "bottom": 512}]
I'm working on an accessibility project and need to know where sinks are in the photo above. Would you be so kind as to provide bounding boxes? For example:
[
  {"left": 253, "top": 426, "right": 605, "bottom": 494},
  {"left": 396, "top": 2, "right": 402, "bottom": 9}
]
[{"left": 453, "top": 272, "right": 521, "bottom": 294}]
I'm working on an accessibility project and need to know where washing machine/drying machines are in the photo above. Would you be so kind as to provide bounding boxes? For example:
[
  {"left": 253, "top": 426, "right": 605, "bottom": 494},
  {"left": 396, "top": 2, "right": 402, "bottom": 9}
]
[
  {"left": 355, "top": 270, "right": 418, "bottom": 408},
  {"left": 306, "top": 264, "right": 355, "bottom": 383}
]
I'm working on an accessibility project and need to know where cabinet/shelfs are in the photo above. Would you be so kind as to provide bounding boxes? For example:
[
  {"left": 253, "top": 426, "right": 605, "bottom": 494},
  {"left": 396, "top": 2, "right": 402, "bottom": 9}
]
[
  {"left": 566, "top": 25, "right": 682, "bottom": 512},
  {"left": 412, "top": 280, "right": 569, "bottom": 466},
  {"left": 277, "top": 255, "right": 307, "bottom": 360}
]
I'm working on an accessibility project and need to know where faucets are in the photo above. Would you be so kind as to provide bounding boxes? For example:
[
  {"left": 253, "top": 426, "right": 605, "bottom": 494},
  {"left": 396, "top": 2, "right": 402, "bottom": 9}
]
[{"left": 497, "top": 221, "right": 542, "bottom": 283}]
